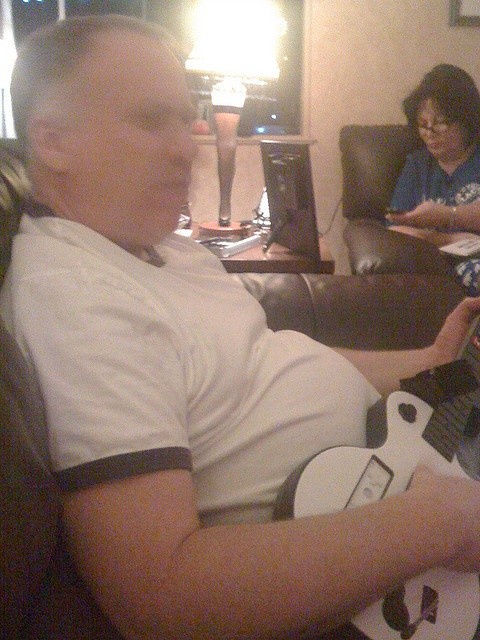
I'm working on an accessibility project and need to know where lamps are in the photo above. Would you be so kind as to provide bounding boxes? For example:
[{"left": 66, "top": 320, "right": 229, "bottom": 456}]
[{"left": 182, "top": 0, "right": 288, "bottom": 238}]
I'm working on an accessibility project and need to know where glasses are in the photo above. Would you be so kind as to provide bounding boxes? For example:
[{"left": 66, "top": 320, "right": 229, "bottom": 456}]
[{"left": 411, "top": 117, "right": 456, "bottom": 139}]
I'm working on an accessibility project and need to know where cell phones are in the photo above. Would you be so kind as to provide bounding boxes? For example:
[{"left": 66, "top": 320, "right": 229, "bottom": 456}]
[{"left": 385, "top": 204, "right": 407, "bottom": 214}]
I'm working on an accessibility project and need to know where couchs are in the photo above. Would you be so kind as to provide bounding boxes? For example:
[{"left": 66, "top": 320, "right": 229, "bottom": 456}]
[
  {"left": 0, "top": 137, "right": 467, "bottom": 640},
  {"left": 339, "top": 124, "right": 465, "bottom": 291}
]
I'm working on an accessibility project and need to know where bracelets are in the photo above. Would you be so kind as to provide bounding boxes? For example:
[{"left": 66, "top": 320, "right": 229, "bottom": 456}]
[{"left": 449, "top": 204, "right": 458, "bottom": 231}]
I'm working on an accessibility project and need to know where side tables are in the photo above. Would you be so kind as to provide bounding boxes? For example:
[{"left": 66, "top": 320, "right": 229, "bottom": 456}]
[{"left": 172, "top": 219, "right": 335, "bottom": 274}]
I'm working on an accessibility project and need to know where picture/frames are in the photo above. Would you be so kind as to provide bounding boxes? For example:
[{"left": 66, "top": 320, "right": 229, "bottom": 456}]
[{"left": 448, "top": 0, "right": 480, "bottom": 27}]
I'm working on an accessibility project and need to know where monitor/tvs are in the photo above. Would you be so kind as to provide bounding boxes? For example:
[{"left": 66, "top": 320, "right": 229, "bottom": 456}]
[{"left": 257, "top": 137, "right": 321, "bottom": 261}]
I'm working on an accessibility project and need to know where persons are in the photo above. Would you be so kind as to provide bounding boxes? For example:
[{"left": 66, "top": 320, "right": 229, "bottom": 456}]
[
  {"left": 1, "top": 15, "right": 480, "bottom": 638},
  {"left": 384, "top": 61, "right": 479, "bottom": 303}
]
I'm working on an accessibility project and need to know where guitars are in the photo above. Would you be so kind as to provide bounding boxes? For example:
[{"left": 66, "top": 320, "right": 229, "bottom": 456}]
[{"left": 272, "top": 315, "right": 480, "bottom": 639}]
